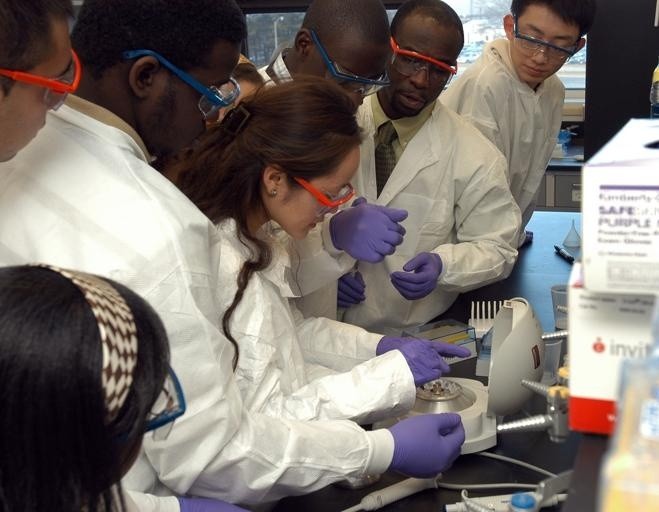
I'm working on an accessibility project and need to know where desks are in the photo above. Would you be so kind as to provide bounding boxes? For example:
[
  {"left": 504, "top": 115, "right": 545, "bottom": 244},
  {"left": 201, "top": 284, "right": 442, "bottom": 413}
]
[{"left": 231, "top": 210, "right": 605, "bottom": 512}]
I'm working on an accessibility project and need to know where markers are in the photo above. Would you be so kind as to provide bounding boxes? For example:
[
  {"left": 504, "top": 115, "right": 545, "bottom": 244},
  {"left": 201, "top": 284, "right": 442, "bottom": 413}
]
[{"left": 553, "top": 244, "right": 574, "bottom": 263}]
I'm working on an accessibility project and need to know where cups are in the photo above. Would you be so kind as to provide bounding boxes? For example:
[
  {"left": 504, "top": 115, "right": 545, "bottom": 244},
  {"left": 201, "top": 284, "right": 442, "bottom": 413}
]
[
  {"left": 551, "top": 284, "right": 573, "bottom": 330},
  {"left": 540, "top": 333, "right": 563, "bottom": 385}
]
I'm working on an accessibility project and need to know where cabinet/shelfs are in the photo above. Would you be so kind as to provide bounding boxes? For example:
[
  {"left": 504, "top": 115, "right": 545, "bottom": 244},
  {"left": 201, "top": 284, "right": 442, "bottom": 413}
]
[{"left": 532, "top": 144, "right": 585, "bottom": 212}]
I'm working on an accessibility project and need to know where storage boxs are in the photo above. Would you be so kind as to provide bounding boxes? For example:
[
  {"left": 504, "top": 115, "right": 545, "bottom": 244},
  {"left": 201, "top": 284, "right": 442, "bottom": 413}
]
[
  {"left": 567, "top": 263, "right": 659, "bottom": 435},
  {"left": 580, "top": 118, "right": 658, "bottom": 295}
]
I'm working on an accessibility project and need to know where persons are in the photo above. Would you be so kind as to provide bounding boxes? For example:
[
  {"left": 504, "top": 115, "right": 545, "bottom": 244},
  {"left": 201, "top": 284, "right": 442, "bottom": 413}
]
[
  {"left": 167, "top": 74, "right": 471, "bottom": 426},
  {"left": 0, "top": 1, "right": 466, "bottom": 512},
  {"left": 255, "top": 1, "right": 408, "bottom": 318},
  {"left": 297, "top": 2, "right": 524, "bottom": 336},
  {"left": 0, "top": 264, "right": 251, "bottom": 511},
  {"left": 1, "top": 0, "right": 81, "bottom": 165},
  {"left": 148, "top": 53, "right": 263, "bottom": 184},
  {"left": 442, "top": 2, "right": 597, "bottom": 217}
]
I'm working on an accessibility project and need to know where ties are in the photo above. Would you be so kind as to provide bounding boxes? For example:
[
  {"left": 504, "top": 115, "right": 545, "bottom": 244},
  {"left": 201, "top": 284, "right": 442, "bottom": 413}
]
[{"left": 372, "top": 120, "right": 398, "bottom": 200}]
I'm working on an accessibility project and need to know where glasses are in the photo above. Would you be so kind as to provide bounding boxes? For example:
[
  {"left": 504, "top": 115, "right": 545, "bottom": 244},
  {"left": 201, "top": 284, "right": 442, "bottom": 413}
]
[
  {"left": 1, "top": 47, "right": 84, "bottom": 112},
  {"left": 284, "top": 169, "right": 354, "bottom": 216},
  {"left": 388, "top": 34, "right": 458, "bottom": 91},
  {"left": 513, "top": 13, "right": 577, "bottom": 62},
  {"left": 124, "top": 47, "right": 243, "bottom": 125},
  {"left": 307, "top": 28, "right": 392, "bottom": 98},
  {"left": 143, "top": 363, "right": 187, "bottom": 443}
]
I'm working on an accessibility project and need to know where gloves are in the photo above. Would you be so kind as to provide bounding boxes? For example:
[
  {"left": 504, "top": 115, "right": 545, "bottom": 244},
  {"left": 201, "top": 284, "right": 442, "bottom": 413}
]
[
  {"left": 335, "top": 272, "right": 369, "bottom": 308},
  {"left": 383, "top": 412, "right": 465, "bottom": 481},
  {"left": 176, "top": 495, "right": 254, "bottom": 512},
  {"left": 389, "top": 252, "right": 443, "bottom": 302},
  {"left": 326, "top": 196, "right": 409, "bottom": 265},
  {"left": 374, "top": 330, "right": 471, "bottom": 388}
]
